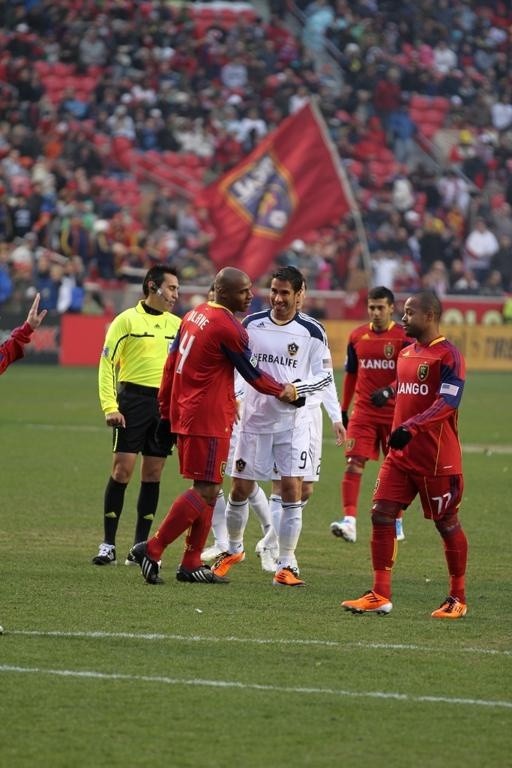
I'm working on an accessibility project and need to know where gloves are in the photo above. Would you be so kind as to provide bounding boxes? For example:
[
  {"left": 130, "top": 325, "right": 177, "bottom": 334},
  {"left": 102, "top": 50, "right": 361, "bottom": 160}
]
[
  {"left": 367, "top": 385, "right": 394, "bottom": 410},
  {"left": 340, "top": 410, "right": 349, "bottom": 434},
  {"left": 155, "top": 417, "right": 178, "bottom": 446},
  {"left": 386, "top": 426, "right": 412, "bottom": 452}
]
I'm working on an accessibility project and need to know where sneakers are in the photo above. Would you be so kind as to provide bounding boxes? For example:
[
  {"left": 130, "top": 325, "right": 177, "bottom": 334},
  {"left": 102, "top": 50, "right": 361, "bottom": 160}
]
[
  {"left": 126, "top": 555, "right": 135, "bottom": 566},
  {"left": 395, "top": 518, "right": 407, "bottom": 545},
  {"left": 92, "top": 542, "right": 118, "bottom": 568},
  {"left": 125, "top": 541, "right": 165, "bottom": 585},
  {"left": 273, "top": 571, "right": 308, "bottom": 588},
  {"left": 429, "top": 595, "right": 468, "bottom": 619},
  {"left": 339, "top": 589, "right": 395, "bottom": 616},
  {"left": 199, "top": 543, "right": 222, "bottom": 562},
  {"left": 254, "top": 538, "right": 276, "bottom": 574},
  {"left": 330, "top": 519, "right": 360, "bottom": 544},
  {"left": 229, "top": 543, "right": 244, "bottom": 560},
  {"left": 175, "top": 564, "right": 231, "bottom": 584},
  {"left": 210, "top": 549, "right": 251, "bottom": 578}
]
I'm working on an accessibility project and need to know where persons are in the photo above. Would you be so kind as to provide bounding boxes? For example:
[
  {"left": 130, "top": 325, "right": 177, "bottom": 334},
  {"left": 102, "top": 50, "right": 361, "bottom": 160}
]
[
  {"left": 191, "top": 3, "right": 318, "bottom": 100},
  {"left": 3, "top": 2, "right": 194, "bottom": 314},
  {"left": 208, "top": 268, "right": 333, "bottom": 588},
  {"left": 255, "top": 274, "right": 347, "bottom": 576},
  {"left": 93, "top": 267, "right": 186, "bottom": 565},
  {"left": 1, "top": 293, "right": 48, "bottom": 373},
  {"left": 315, "top": 0, "right": 510, "bottom": 169},
  {"left": 127, "top": 267, "right": 306, "bottom": 584},
  {"left": 352, "top": 166, "right": 511, "bottom": 295},
  {"left": 340, "top": 287, "right": 469, "bottom": 616},
  {"left": 201, "top": 284, "right": 279, "bottom": 562},
  {"left": 331, "top": 286, "right": 407, "bottom": 545}
]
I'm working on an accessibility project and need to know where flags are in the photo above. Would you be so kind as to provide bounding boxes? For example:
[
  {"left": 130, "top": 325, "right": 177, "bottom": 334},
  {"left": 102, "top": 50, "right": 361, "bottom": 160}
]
[
  {"left": 193, "top": 102, "right": 374, "bottom": 286},
  {"left": 196, "top": 98, "right": 373, "bottom": 312}
]
